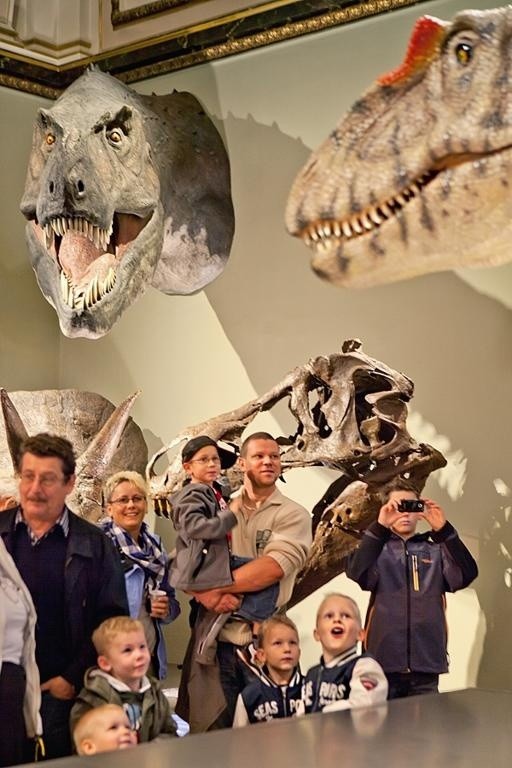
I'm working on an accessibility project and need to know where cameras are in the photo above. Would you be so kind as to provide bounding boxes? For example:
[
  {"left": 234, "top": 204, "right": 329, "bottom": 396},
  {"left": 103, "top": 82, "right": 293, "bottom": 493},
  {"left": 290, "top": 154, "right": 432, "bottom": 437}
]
[{"left": 396, "top": 501, "right": 426, "bottom": 511}]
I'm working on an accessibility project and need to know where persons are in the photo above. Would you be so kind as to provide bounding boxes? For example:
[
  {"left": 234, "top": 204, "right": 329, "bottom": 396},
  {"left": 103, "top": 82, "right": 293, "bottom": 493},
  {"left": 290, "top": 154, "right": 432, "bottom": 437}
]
[
  {"left": 0, "top": 432, "right": 131, "bottom": 761},
  {"left": 175, "top": 431, "right": 313, "bottom": 735},
  {"left": 72, "top": 703, "right": 135, "bottom": 757},
  {"left": 344, "top": 485, "right": 479, "bottom": 700},
  {"left": 232, "top": 615, "right": 305, "bottom": 730},
  {"left": 95, "top": 470, "right": 181, "bottom": 682},
  {"left": 295, "top": 592, "right": 388, "bottom": 718},
  {"left": 168, "top": 434, "right": 280, "bottom": 678},
  {"left": 0, "top": 533, "right": 42, "bottom": 768},
  {"left": 69, "top": 616, "right": 180, "bottom": 746}
]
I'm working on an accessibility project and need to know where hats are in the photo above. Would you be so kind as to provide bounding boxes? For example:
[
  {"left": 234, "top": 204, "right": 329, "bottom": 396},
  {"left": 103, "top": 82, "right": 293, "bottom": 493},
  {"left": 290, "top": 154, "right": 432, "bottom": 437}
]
[{"left": 182, "top": 432, "right": 219, "bottom": 460}]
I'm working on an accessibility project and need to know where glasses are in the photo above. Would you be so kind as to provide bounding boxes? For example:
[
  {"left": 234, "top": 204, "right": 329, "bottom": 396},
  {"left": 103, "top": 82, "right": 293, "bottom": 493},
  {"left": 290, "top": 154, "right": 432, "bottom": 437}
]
[
  {"left": 188, "top": 456, "right": 222, "bottom": 464},
  {"left": 109, "top": 495, "right": 147, "bottom": 503}
]
